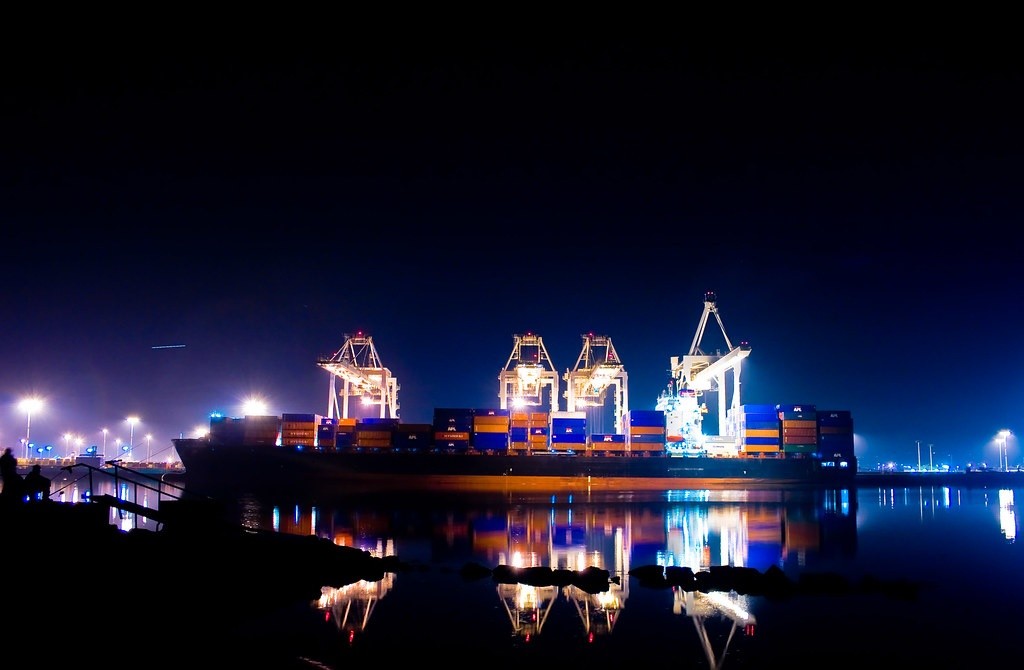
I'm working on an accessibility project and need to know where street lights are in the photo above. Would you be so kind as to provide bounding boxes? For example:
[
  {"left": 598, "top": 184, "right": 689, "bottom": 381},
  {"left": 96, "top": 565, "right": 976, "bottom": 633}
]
[
  {"left": 116, "top": 438, "right": 121, "bottom": 458},
  {"left": 76, "top": 438, "right": 81, "bottom": 461},
  {"left": 47, "top": 446, "right": 53, "bottom": 460},
  {"left": 19, "top": 400, "right": 38, "bottom": 460},
  {"left": 19, "top": 437, "right": 26, "bottom": 456},
  {"left": 998, "top": 429, "right": 1011, "bottom": 471},
  {"left": 29, "top": 444, "right": 34, "bottom": 459},
  {"left": 995, "top": 437, "right": 1005, "bottom": 471},
  {"left": 926, "top": 442, "right": 934, "bottom": 473},
  {"left": 64, "top": 435, "right": 70, "bottom": 458},
  {"left": 37, "top": 449, "right": 43, "bottom": 461},
  {"left": 146, "top": 434, "right": 152, "bottom": 464},
  {"left": 127, "top": 417, "right": 139, "bottom": 461},
  {"left": 915, "top": 439, "right": 926, "bottom": 473},
  {"left": 103, "top": 428, "right": 107, "bottom": 458}
]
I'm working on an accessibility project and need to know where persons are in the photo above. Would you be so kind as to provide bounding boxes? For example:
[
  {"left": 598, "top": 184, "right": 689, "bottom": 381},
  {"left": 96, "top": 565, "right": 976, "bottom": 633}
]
[{"left": 0, "top": 448, "right": 53, "bottom": 506}]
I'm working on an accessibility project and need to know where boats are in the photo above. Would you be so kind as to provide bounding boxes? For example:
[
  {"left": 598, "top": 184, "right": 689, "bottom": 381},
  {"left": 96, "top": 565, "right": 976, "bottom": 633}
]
[
  {"left": 156, "top": 492, "right": 858, "bottom": 670},
  {"left": 167, "top": 289, "right": 864, "bottom": 505}
]
